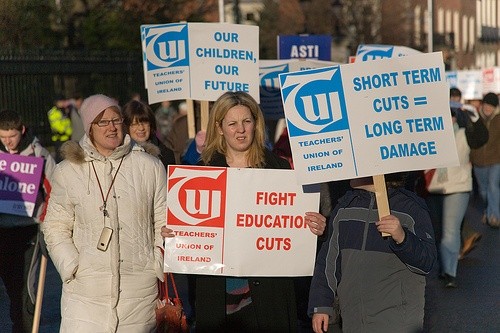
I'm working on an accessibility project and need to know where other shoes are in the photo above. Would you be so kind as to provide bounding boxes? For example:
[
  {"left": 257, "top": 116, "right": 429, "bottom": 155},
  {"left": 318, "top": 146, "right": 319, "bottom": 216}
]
[
  {"left": 461, "top": 231, "right": 482, "bottom": 255},
  {"left": 481, "top": 209, "right": 500, "bottom": 226},
  {"left": 445, "top": 276, "right": 457, "bottom": 287}
]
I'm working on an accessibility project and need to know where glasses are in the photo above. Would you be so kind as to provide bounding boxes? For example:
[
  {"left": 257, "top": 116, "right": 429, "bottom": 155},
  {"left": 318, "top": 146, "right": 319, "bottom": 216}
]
[
  {"left": 131, "top": 120, "right": 150, "bottom": 127},
  {"left": 92, "top": 118, "right": 124, "bottom": 127}
]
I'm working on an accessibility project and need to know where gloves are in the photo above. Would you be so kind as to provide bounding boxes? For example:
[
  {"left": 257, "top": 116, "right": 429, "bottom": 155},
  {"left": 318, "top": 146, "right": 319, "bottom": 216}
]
[{"left": 464, "top": 104, "right": 480, "bottom": 123}]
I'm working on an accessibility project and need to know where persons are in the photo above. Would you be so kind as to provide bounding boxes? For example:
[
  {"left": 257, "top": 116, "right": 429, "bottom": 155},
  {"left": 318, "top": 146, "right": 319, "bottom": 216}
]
[
  {"left": 274, "top": 118, "right": 295, "bottom": 170},
  {"left": 122, "top": 100, "right": 176, "bottom": 174},
  {"left": 470, "top": 92, "right": 500, "bottom": 227},
  {"left": 449, "top": 87, "right": 464, "bottom": 109},
  {"left": 307, "top": 173, "right": 439, "bottom": 333},
  {"left": 423, "top": 104, "right": 489, "bottom": 288},
  {"left": 179, "top": 130, "right": 208, "bottom": 166},
  {"left": 153, "top": 100, "right": 179, "bottom": 142},
  {"left": 161, "top": 91, "right": 326, "bottom": 333},
  {"left": 162, "top": 102, "right": 189, "bottom": 165},
  {"left": 67, "top": 94, "right": 87, "bottom": 144},
  {"left": 40, "top": 94, "right": 168, "bottom": 333},
  {"left": 468, "top": 100, "right": 483, "bottom": 112},
  {"left": 0, "top": 110, "right": 56, "bottom": 333},
  {"left": 47, "top": 95, "right": 74, "bottom": 164}
]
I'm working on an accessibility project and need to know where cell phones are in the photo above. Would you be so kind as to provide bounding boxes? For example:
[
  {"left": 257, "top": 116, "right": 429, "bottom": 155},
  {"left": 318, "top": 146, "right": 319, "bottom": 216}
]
[{"left": 97, "top": 227, "right": 113, "bottom": 251}]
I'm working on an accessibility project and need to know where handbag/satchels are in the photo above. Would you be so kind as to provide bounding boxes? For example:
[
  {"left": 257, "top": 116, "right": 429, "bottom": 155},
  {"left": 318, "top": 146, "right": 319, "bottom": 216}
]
[{"left": 153, "top": 245, "right": 187, "bottom": 333}]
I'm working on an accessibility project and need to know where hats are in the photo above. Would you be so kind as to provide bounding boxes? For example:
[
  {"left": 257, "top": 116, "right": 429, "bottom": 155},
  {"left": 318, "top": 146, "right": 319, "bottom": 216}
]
[
  {"left": 79, "top": 94, "right": 122, "bottom": 137},
  {"left": 483, "top": 93, "right": 498, "bottom": 107}
]
